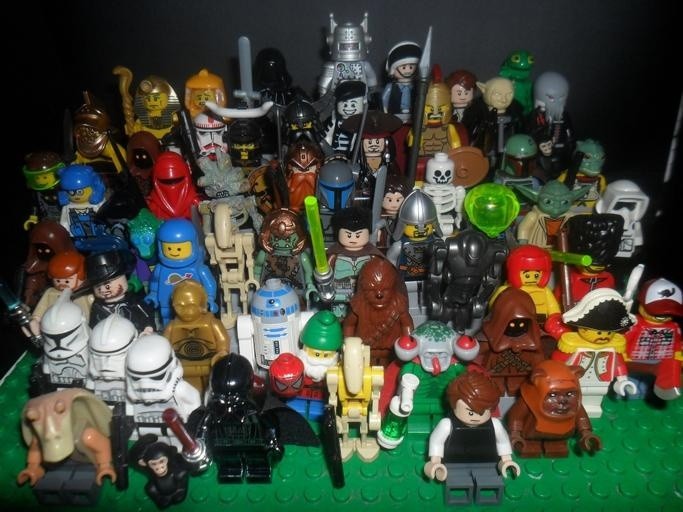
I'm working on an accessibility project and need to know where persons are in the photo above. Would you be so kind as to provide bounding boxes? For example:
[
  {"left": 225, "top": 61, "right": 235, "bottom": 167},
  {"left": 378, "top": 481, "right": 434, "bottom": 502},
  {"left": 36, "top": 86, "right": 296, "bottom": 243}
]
[
  {"left": 326, "top": 40, "right": 524, "bottom": 184},
  {"left": 124, "top": 80, "right": 179, "bottom": 138},
  {"left": 23, "top": 219, "right": 230, "bottom": 395},
  {"left": 311, "top": 182, "right": 447, "bottom": 321},
  {"left": 287, "top": 312, "right": 346, "bottom": 419},
  {"left": 423, "top": 374, "right": 520, "bottom": 506},
  {"left": 488, "top": 246, "right": 683, "bottom": 419},
  {"left": 0, "top": 8, "right": 683, "bottom": 512}
]
